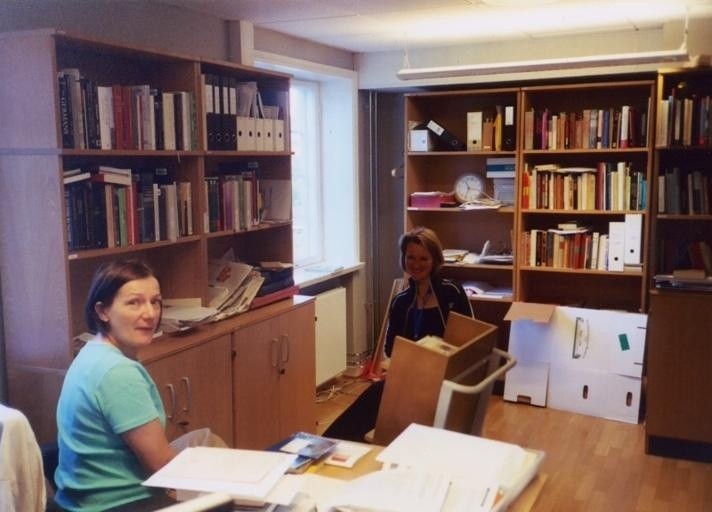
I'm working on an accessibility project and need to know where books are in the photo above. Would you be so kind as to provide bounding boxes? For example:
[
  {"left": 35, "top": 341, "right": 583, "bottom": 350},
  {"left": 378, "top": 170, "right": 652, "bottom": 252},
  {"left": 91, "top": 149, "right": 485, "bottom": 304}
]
[
  {"left": 203, "top": 170, "right": 264, "bottom": 233},
  {"left": 248, "top": 263, "right": 299, "bottom": 309},
  {"left": 462, "top": 279, "right": 512, "bottom": 300},
  {"left": 522, "top": 88, "right": 712, "bottom": 291},
  {"left": 59, "top": 67, "right": 198, "bottom": 247},
  {"left": 229, "top": 431, "right": 338, "bottom": 512}
]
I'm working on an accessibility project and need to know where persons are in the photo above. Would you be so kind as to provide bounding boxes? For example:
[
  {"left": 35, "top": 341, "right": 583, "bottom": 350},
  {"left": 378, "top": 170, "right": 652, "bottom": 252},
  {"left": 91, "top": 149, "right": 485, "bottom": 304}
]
[
  {"left": 383, "top": 226, "right": 475, "bottom": 360},
  {"left": 53, "top": 259, "right": 229, "bottom": 512}
]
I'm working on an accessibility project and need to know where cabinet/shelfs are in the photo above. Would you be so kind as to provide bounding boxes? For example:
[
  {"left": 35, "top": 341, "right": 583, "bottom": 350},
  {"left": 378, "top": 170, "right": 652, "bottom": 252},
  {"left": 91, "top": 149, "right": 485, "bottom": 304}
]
[
  {"left": 403, "top": 87, "right": 521, "bottom": 395},
  {"left": 0, "top": 28, "right": 235, "bottom": 449},
  {"left": 197, "top": 56, "right": 316, "bottom": 448},
  {"left": 644, "top": 66, "right": 712, "bottom": 454},
  {"left": 515, "top": 80, "right": 656, "bottom": 313}
]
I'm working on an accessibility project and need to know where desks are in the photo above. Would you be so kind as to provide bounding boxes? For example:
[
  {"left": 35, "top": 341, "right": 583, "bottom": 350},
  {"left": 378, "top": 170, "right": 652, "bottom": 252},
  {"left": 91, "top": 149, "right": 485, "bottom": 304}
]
[{"left": 231, "top": 439, "right": 548, "bottom": 512}]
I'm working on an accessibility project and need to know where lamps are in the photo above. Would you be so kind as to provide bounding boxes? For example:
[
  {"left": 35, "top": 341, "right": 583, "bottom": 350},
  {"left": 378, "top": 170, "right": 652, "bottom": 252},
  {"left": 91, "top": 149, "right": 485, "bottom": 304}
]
[{"left": 396, "top": 17, "right": 690, "bottom": 79}]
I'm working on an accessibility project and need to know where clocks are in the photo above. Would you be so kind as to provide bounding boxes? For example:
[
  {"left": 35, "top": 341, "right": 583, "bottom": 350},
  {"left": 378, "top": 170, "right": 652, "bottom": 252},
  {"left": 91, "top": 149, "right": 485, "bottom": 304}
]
[{"left": 454, "top": 172, "right": 485, "bottom": 203}]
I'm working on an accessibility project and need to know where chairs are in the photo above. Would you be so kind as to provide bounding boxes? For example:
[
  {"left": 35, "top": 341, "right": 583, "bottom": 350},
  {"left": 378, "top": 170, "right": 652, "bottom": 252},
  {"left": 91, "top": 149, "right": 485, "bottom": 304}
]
[{"left": 363, "top": 347, "right": 518, "bottom": 446}]
